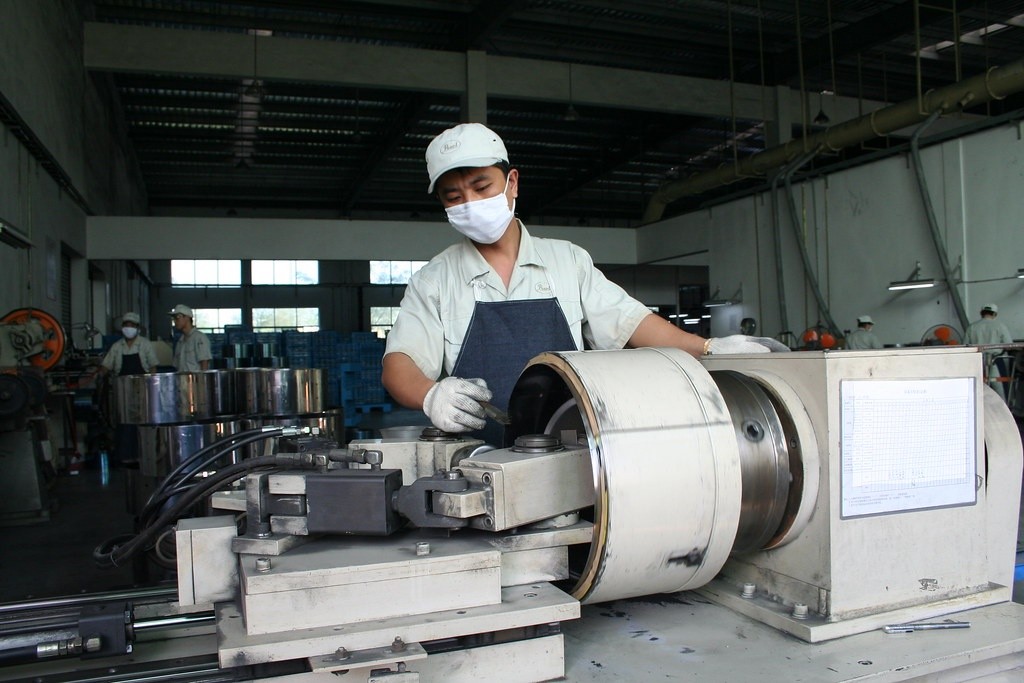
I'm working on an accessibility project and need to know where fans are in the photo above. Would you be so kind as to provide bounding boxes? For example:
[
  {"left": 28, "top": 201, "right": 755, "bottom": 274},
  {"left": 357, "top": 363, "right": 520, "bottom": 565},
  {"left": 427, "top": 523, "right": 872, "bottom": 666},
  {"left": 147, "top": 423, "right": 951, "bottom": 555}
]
[
  {"left": 796, "top": 326, "right": 839, "bottom": 351},
  {"left": 920, "top": 324, "right": 963, "bottom": 345}
]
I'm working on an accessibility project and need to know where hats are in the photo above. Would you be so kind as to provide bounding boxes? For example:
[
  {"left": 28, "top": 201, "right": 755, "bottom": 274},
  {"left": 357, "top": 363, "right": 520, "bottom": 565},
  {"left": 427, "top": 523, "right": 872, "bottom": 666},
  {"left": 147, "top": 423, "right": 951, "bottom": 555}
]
[
  {"left": 121, "top": 312, "right": 140, "bottom": 324},
  {"left": 426, "top": 123, "right": 509, "bottom": 193},
  {"left": 981, "top": 304, "right": 998, "bottom": 313},
  {"left": 166, "top": 305, "right": 194, "bottom": 317},
  {"left": 857, "top": 315, "right": 875, "bottom": 323}
]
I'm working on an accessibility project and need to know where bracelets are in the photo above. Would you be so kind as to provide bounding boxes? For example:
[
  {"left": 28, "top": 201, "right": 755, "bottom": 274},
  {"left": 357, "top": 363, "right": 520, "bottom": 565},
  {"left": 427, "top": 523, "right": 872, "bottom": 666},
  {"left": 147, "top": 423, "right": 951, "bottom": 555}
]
[{"left": 704, "top": 338, "right": 712, "bottom": 354}]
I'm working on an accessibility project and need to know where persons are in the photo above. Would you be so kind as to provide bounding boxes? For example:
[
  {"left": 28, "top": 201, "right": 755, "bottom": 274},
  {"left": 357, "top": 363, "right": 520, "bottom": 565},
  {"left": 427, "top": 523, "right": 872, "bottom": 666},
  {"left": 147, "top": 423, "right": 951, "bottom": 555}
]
[
  {"left": 964, "top": 303, "right": 1013, "bottom": 404},
  {"left": 98, "top": 304, "right": 213, "bottom": 463},
  {"left": 845, "top": 315, "right": 883, "bottom": 349},
  {"left": 382, "top": 122, "right": 792, "bottom": 452}
]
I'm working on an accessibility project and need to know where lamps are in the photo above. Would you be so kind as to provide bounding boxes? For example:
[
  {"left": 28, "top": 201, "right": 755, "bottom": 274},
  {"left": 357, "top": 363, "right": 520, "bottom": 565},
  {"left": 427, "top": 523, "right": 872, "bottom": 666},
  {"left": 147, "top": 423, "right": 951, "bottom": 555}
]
[
  {"left": 703, "top": 286, "right": 744, "bottom": 307},
  {"left": 562, "top": 54, "right": 578, "bottom": 120},
  {"left": 887, "top": 262, "right": 936, "bottom": 290},
  {"left": 235, "top": 87, "right": 253, "bottom": 173},
  {"left": 67, "top": 321, "right": 99, "bottom": 340},
  {"left": 812, "top": 67, "right": 830, "bottom": 124},
  {"left": 244, "top": 29, "right": 265, "bottom": 98}
]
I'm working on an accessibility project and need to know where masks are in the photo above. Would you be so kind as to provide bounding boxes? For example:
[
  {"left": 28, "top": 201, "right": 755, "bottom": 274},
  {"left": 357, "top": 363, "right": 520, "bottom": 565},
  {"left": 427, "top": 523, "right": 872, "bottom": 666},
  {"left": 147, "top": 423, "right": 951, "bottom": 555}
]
[
  {"left": 122, "top": 327, "right": 140, "bottom": 338},
  {"left": 444, "top": 173, "right": 516, "bottom": 244}
]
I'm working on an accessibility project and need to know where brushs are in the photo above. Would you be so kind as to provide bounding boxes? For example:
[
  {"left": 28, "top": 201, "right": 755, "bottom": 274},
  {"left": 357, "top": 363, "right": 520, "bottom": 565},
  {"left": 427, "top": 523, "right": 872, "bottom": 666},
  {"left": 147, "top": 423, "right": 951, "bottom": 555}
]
[{"left": 475, "top": 399, "right": 511, "bottom": 426}]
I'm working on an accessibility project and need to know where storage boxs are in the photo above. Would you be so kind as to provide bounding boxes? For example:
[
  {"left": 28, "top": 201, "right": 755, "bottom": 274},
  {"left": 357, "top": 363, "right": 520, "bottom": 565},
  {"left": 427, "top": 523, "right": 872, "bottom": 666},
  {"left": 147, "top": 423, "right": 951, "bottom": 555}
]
[{"left": 207, "top": 325, "right": 391, "bottom": 426}]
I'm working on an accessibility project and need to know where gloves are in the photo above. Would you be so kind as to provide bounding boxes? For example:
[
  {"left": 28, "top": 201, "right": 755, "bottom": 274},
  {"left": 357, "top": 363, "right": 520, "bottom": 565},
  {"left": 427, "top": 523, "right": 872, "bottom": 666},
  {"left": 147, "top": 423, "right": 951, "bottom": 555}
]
[
  {"left": 703, "top": 334, "right": 790, "bottom": 354},
  {"left": 423, "top": 377, "right": 492, "bottom": 432}
]
